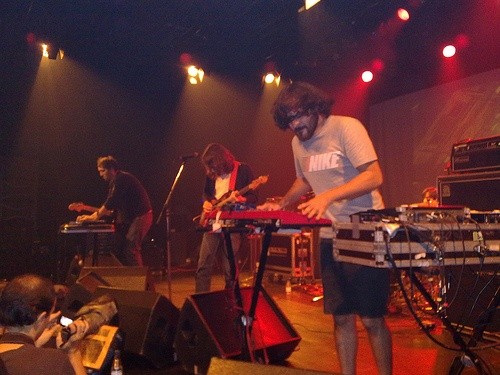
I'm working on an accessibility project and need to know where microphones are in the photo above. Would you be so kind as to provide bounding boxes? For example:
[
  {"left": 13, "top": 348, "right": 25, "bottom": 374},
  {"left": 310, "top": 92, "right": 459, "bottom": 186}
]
[{"left": 178, "top": 152, "right": 198, "bottom": 161}]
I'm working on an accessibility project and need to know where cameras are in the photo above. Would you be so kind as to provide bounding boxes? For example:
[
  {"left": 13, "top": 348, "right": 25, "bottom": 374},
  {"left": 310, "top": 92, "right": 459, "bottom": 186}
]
[{"left": 57, "top": 315, "right": 79, "bottom": 333}]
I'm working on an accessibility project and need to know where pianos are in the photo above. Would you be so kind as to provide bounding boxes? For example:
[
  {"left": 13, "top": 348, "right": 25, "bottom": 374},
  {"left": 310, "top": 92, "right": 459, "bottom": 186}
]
[{"left": 203, "top": 208, "right": 333, "bottom": 364}]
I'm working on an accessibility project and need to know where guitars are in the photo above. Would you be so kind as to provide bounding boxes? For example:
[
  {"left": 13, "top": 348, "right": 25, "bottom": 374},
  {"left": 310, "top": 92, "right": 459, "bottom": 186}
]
[
  {"left": 69, "top": 202, "right": 115, "bottom": 224},
  {"left": 199, "top": 174, "right": 269, "bottom": 229}
]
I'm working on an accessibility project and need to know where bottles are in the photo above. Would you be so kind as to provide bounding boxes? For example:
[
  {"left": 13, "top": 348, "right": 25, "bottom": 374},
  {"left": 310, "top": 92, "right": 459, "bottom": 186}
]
[
  {"left": 111, "top": 350, "right": 123, "bottom": 375},
  {"left": 286, "top": 278, "right": 291, "bottom": 293}
]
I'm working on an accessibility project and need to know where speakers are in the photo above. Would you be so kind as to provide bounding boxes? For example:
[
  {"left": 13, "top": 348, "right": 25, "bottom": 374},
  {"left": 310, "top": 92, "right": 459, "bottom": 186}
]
[
  {"left": 92, "top": 285, "right": 180, "bottom": 365},
  {"left": 170, "top": 282, "right": 303, "bottom": 373},
  {"left": 206, "top": 357, "right": 334, "bottom": 375},
  {"left": 436, "top": 172, "right": 500, "bottom": 343}
]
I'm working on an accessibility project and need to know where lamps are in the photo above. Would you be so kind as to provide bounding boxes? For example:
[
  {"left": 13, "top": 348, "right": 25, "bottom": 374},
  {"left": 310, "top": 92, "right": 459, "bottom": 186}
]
[
  {"left": 188, "top": 63, "right": 205, "bottom": 84},
  {"left": 265, "top": 67, "right": 281, "bottom": 87}
]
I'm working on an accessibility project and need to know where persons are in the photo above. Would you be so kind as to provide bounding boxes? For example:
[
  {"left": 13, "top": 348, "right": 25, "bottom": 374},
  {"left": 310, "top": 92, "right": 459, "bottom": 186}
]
[
  {"left": 194, "top": 142, "right": 266, "bottom": 293},
  {"left": 76, "top": 156, "right": 153, "bottom": 266},
  {"left": 256, "top": 80, "right": 393, "bottom": 375},
  {"left": 0, "top": 274, "right": 90, "bottom": 375}
]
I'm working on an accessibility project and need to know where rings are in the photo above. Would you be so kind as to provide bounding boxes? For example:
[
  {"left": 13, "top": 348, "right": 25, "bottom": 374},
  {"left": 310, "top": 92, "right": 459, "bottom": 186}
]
[{"left": 80, "top": 331, "right": 86, "bottom": 333}]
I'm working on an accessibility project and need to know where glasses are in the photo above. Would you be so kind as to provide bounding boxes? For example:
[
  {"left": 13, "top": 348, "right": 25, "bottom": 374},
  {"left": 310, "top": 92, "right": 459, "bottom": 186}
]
[{"left": 287, "top": 108, "right": 309, "bottom": 123}]
